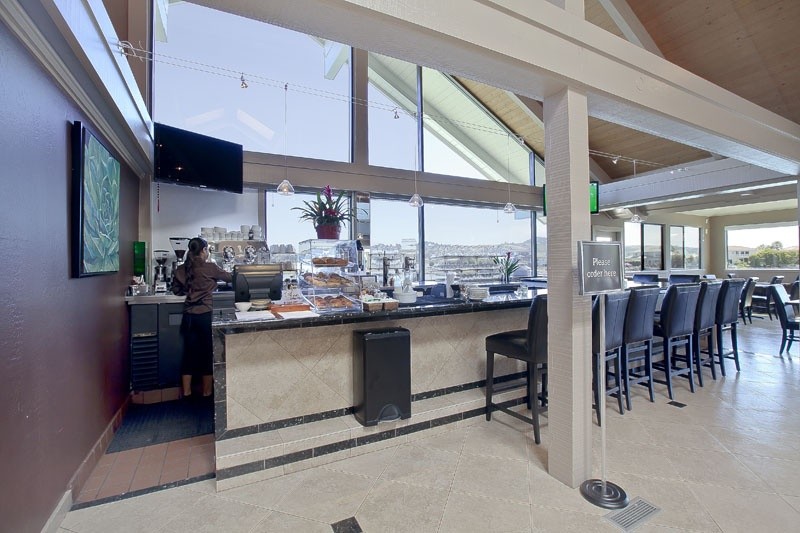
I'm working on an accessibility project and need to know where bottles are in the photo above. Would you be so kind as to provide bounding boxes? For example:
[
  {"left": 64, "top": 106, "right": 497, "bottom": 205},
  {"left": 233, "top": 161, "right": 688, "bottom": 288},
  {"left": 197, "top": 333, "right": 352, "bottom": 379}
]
[
  {"left": 404, "top": 268, "right": 410, "bottom": 278},
  {"left": 410, "top": 270, "right": 418, "bottom": 282},
  {"left": 375, "top": 254, "right": 401, "bottom": 267},
  {"left": 403, "top": 276, "right": 413, "bottom": 293},
  {"left": 257, "top": 247, "right": 269, "bottom": 264}
]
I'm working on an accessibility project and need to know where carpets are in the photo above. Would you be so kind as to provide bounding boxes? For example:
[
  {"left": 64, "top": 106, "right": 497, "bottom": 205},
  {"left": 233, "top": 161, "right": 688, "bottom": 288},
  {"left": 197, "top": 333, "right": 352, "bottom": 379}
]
[{"left": 105, "top": 396, "right": 215, "bottom": 454}]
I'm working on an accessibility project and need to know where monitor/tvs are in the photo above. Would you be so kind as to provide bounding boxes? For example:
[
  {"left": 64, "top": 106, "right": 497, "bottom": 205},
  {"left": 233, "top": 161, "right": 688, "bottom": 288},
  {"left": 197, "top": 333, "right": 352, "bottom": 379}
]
[
  {"left": 543, "top": 181, "right": 599, "bottom": 216},
  {"left": 153, "top": 122, "right": 243, "bottom": 193},
  {"left": 234, "top": 264, "right": 282, "bottom": 312}
]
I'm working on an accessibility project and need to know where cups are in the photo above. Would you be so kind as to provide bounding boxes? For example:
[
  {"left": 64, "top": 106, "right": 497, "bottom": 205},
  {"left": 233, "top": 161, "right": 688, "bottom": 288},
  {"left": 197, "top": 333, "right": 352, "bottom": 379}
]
[
  {"left": 214, "top": 227, "right": 226, "bottom": 233},
  {"left": 241, "top": 225, "right": 249, "bottom": 239},
  {"left": 252, "top": 225, "right": 261, "bottom": 239},
  {"left": 198, "top": 232, "right": 213, "bottom": 240},
  {"left": 658, "top": 270, "right": 670, "bottom": 289},
  {"left": 394, "top": 280, "right": 402, "bottom": 292},
  {"left": 270, "top": 244, "right": 293, "bottom": 254},
  {"left": 213, "top": 232, "right": 232, "bottom": 240},
  {"left": 368, "top": 283, "right": 387, "bottom": 299},
  {"left": 231, "top": 231, "right": 243, "bottom": 241},
  {"left": 281, "top": 288, "right": 303, "bottom": 303},
  {"left": 520, "top": 283, "right": 528, "bottom": 299},
  {"left": 201, "top": 228, "right": 214, "bottom": 234}
]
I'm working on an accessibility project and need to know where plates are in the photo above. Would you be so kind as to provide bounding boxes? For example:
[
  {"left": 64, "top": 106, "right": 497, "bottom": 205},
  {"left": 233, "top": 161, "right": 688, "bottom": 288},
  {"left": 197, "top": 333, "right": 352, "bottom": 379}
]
[{"left": 470, "top": 297, "right": 483, "bottom": 300}]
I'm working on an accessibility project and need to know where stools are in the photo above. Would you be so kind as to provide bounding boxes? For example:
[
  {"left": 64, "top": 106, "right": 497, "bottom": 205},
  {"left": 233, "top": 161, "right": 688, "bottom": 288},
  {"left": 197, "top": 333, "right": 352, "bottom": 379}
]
[{"left": 486, "top": 274, "right": 745, "bottom": 444}]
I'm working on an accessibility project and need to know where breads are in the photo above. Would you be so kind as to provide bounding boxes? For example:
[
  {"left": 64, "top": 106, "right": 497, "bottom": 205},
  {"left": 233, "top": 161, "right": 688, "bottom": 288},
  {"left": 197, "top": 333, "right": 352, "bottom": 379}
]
[
  {"left": 303, "top": 271, "right": 342, "bottom": 288},
  {"left": 312, "top": 295, "right": 353, "bottom": 307},
  {"left": 312, "top": 256, "right": 348, "bottom": 265}
]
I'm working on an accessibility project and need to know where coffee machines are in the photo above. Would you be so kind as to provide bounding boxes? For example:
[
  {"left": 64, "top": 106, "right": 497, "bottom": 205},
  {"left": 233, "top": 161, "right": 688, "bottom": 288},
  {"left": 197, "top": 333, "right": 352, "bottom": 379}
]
[
  {"left": 169, "top": 237, "right": 191, "bottom": 294},
  {"left": 153, "top": 250, "right": 170, "bottom": 293}
]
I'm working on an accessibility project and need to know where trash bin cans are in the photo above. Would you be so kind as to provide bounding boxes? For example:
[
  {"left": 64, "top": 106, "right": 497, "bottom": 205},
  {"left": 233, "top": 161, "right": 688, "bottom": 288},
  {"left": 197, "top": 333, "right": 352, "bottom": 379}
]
[{"left": 354, "top": 327, "right": 411, "bottom": 427}]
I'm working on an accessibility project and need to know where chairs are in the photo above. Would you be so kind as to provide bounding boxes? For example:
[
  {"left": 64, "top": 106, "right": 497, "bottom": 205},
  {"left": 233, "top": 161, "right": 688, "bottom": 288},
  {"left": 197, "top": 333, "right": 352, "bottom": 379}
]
[{"left": 703, "top": 273, "right": 800, "bottom": 355}]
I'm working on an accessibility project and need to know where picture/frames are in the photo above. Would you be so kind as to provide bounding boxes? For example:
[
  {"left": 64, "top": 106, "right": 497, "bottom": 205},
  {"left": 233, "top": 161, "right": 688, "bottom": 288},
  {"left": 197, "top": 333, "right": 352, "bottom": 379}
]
[{"left": 72, "top": 121, "right": 120, "bottom": 278}]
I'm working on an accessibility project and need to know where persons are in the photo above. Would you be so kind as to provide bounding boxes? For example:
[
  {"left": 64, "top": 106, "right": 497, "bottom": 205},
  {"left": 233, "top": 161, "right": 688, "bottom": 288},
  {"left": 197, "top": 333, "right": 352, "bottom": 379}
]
[{"left": 169, "top": 238, "right": 232, "bottom": 407}]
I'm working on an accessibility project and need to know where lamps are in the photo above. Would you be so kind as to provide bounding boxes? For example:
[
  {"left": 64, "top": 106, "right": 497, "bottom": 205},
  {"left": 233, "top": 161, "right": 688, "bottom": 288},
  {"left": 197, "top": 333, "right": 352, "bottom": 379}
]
[
  {"left": 504, "top": 133, "right": 517, "bottom": 214},
  {"left": 240, "top": 72, "right": 247, "bottom": 88},
  {"left": 519, "top": 137, "right": 525, "bottom": 146},
  {"left": 409, "top": 112, "right": 424, "bottom": 207},
  {"left": 612, "top": 156, "right": 621, "bottom": 165},
  {"left": 392, "top": 107, "right": 400, "bottom": 118},
  {"left": 631, "top": 160, "right": 641, "bottom": 223},
  {"left": 276, "top": 83, "right": 295, "bottom": 196}
]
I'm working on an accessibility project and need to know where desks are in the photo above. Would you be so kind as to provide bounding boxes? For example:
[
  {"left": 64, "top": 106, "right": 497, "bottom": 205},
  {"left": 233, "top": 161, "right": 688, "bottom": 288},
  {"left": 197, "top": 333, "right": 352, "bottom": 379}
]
[{"left": 742, "top": 281, "right": 791, "bottom": 314}]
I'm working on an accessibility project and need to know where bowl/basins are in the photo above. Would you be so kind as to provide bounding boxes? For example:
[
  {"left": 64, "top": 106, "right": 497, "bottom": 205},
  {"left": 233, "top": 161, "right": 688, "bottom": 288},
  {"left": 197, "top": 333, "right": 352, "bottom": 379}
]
[
  {"left": 235, "top": 302, "right": 252, "bottom": 312},
  {"left": 470, "top": 287, "right": 489, "bottom": 298}
]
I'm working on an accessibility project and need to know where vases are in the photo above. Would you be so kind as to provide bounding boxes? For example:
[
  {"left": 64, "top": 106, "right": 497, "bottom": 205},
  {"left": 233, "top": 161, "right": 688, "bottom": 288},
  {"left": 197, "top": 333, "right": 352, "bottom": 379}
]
[
  {"left": 503, "top": 273, "right": 510, "bottom": 285},
  {"left": 316, "top": 225, "right": 341, "bottom": 240}
]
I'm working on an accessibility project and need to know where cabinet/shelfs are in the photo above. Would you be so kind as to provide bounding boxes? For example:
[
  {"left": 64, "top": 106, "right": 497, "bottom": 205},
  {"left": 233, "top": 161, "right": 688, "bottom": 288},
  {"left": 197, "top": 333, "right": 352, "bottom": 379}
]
[
  {"left": 269, "top": 252, "right": 299, "bottom": 290},
  {"left": 298, "top": 238, "right": 363, "bottom": 313}
]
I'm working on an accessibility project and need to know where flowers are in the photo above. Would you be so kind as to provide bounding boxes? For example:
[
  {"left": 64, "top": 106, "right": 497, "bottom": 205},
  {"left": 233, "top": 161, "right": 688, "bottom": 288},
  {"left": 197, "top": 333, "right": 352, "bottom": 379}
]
[
  {"left": 493, "top": 252, "right": 520, "bottom": 284},
  {"left": 290, "top": 185, "right": 369, "bottom": 229}
]
